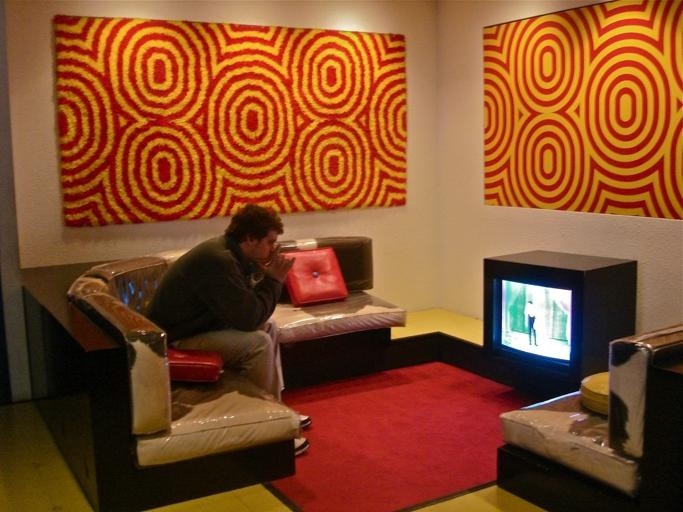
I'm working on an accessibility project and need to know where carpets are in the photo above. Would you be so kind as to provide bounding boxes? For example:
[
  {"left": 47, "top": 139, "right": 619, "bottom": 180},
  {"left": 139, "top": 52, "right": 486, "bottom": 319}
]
[{"left": 258, "top": 361, "right": 532, "bottom": 512}]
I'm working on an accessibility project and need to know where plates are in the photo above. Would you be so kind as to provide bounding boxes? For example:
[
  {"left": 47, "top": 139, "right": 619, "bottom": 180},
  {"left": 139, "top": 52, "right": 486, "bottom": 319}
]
[{"left": 579, "top": 370, "right": 613, "bottom": 417}]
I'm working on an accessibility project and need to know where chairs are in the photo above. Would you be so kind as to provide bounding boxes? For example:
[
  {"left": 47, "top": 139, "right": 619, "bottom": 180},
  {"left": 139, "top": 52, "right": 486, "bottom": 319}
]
[{"left": 496, "top": 322, "right": 683, "bottom": 511}]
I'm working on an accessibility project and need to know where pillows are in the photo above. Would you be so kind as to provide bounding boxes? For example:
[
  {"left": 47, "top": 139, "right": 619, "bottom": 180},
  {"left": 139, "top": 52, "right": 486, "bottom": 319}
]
[
  {"left": 577, "top": 371, "right": 614, "bottom": 415},
  {"left": 278, "top": 243, "right": 349, "bottom": 306},
  {"left": 165, "top": 346, "right": 225, "bottom": 384}
]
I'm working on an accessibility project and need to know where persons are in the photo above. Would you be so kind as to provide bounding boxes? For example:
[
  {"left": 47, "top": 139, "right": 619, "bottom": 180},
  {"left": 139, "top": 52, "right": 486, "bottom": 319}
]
[
  {"left": 145, "top": 203, "right": 312, "bottom": 456},
  {"left": 526, "top": 301, "right": 538, "bottom": 346}
]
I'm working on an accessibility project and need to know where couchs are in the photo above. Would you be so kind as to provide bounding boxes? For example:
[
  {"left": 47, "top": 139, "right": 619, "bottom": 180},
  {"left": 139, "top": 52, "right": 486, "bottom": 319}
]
[{"left": 20, "top": 235, "right": 406, "bottom": 511}]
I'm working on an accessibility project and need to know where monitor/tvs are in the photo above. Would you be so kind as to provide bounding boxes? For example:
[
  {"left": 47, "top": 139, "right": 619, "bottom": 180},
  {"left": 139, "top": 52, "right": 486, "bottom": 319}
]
[{"left": 483, "top": 249, "right": 638, "bottom": 394}]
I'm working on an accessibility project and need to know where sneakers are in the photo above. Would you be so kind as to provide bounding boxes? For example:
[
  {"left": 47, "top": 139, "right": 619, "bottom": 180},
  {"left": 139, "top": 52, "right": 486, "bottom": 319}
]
[
  {"left": 298, "top": 414, "right": 312, "bottom": 429},
  {"left": 293, "top": 437, "right": 310, "bottom": 456}
]
